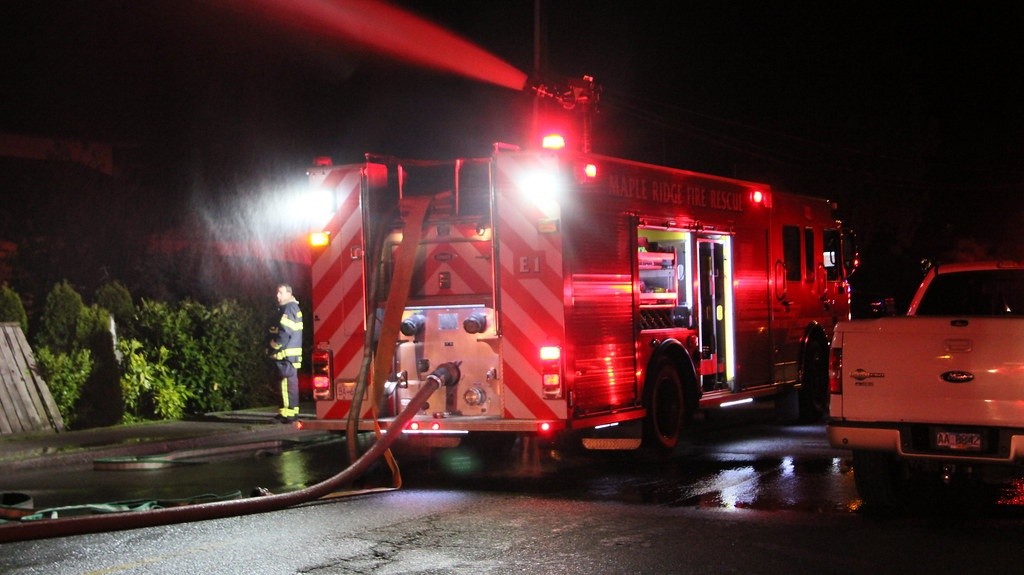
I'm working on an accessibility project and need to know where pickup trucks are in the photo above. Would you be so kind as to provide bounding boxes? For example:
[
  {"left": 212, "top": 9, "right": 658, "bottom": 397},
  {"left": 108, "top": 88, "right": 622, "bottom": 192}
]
[{"left": 827, "top": 260, "right": 1024, "bottom": 510}]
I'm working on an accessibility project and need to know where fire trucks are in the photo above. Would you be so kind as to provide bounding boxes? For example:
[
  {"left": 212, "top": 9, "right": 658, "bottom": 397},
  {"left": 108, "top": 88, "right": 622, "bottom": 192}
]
[{"left": 293, "top": 67, "right": 861, "bottom": 450}]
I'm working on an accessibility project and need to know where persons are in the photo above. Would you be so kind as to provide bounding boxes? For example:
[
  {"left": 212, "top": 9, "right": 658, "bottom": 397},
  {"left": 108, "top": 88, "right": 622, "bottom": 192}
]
[{"left": 266, "top": 280, "right": 304, "bottom": 423}]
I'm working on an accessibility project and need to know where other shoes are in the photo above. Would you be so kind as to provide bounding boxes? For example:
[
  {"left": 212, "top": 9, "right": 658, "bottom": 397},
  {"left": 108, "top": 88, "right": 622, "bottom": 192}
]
[{"left": 268, "top": 414, "right": 298, "bottom": 424}]
